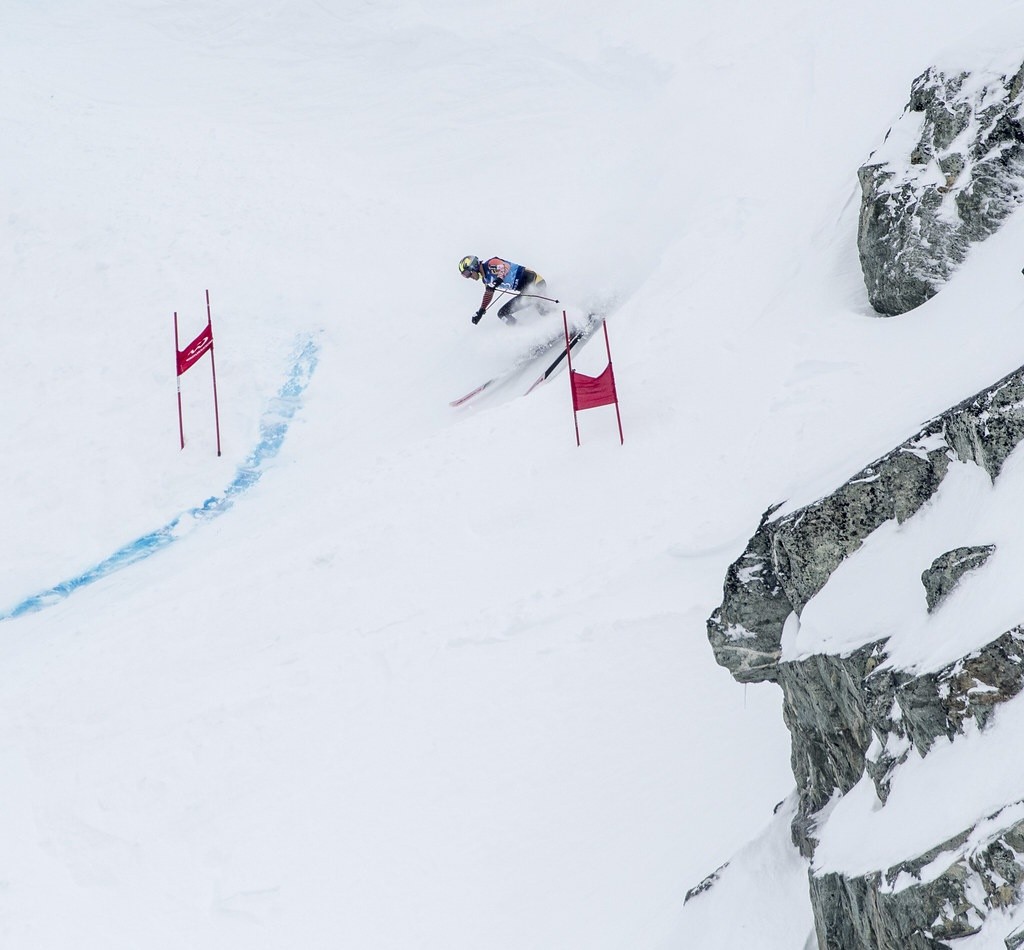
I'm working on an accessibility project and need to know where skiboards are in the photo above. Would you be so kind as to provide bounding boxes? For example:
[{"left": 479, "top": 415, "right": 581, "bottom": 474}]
[{"left": 449, "top": 310, "right": 603, "bottom": 408}]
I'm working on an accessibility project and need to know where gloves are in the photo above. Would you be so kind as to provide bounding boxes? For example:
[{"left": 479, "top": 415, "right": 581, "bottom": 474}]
[{"left": 472, "top": 308, "right": 485, "bottom": 324}]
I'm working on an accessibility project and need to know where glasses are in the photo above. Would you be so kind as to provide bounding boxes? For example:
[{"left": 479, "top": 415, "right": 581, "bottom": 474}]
[{"left": 460, "top": 268, "right": 471, "bottom": 278}]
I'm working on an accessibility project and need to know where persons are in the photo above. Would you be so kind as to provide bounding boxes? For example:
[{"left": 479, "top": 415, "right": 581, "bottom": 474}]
[{"left": 459, "top": 256, "right": 560, "bottom": 326}]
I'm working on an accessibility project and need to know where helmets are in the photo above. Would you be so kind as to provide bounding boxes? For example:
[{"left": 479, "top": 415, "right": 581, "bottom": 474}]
[{"left": 458, "top": 255, "right": 478, "bottom": 279}]
